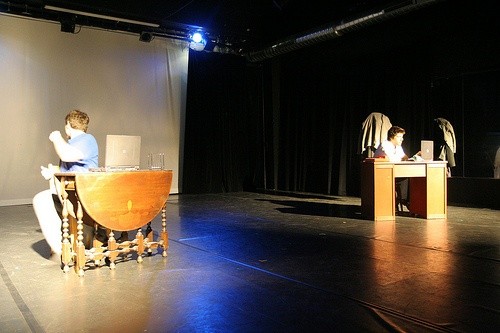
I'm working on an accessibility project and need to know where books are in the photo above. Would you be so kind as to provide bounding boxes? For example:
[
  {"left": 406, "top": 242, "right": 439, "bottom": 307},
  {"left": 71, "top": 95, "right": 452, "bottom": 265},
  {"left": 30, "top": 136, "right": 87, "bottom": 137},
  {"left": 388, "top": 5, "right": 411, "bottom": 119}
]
[{"left": 366, "top": 158, "right": 390, "bottom": 162}]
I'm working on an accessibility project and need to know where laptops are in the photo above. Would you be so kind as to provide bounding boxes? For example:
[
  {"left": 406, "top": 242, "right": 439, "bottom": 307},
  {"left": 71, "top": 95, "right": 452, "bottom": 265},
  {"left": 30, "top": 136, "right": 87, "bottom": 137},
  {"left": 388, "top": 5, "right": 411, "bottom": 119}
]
[
  {"left": 88, "top": 135, "right": 141, "bottom": 172},
  {"left": 407, "top": 140, "right": 433, "bottom": 161}
]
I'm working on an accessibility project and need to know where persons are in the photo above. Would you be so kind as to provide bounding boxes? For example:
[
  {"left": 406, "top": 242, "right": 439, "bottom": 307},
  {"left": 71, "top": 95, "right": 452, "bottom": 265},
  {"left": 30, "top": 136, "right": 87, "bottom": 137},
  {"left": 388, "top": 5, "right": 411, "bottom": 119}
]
[
  {"left": 373, "top": 126, "right": 409, "bottom": 216},
  {"left": 32, "top": 110, "right": 98, "bottom": 266}
]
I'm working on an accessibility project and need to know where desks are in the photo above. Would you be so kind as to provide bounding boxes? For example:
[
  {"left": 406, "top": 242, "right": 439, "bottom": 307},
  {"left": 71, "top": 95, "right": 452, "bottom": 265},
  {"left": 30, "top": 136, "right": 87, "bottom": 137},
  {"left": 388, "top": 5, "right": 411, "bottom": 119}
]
[
  {"left": 54, "top": 169, "right": 173, "bottom": 277},
  {"left": 362, "top": 161, "right": 448, "bottom": 221}
]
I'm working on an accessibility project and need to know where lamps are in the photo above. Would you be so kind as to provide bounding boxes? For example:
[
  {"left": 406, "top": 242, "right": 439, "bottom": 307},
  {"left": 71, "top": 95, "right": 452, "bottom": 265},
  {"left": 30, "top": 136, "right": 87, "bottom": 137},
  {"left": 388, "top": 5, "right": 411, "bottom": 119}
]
[
  {"left": 140, "top": 30, "right": 156, "bottom": 43},
  {"left": 60, "top": 20, "right": 81, "bottom": 34}
]
